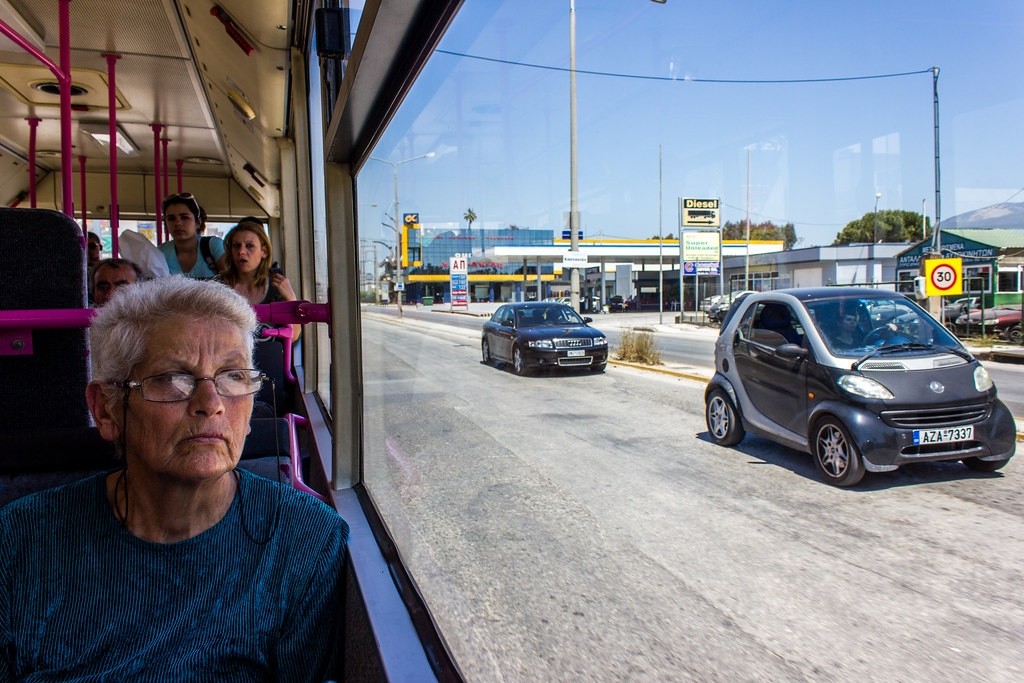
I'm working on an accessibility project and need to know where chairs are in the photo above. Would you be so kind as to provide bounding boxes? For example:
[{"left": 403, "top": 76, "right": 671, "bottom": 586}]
[
  {"left": 0, "top": 206, "right": 98, "bottom": 477},
  {"left": 757, "top": 303, "right": 801, "bottom": 348}
]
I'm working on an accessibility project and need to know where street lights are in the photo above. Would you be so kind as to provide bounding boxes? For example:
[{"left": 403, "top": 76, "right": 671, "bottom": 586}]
[{"left": 370, "top": 152, "right": 436, "bottom": 316}]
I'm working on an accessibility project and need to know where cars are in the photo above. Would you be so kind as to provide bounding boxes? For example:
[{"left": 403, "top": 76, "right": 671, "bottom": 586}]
[
  {"left": 700, "top": 295, "right": 726, "bottom": 314},
  {"left": 482, "top": 301, "right": 608, "bottom": 376},
  {"left": 709, "top": 290, "right": 759, "bottom": 323},
  {"left": 705, "top": 287, "right": 1017, "bottom": 488},
  {"left": 866, "top": 298, "right": 1023, "bottom": 344}
]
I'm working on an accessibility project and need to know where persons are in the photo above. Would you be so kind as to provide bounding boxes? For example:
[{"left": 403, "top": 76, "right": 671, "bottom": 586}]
[
  {"left": 832, "top": 309, "right": 898, "bottom": 349},
  {"left": 152, "top": 193, "right": 233, "bottom": 283},
  {"left": 211, "top": 221, "right": 303, "bottom": 350},
  {"left": 238, "top": 216, "right": 264, "bottom": 228},
  {"left": 0, "top": 273, "right": 352, "bottom": 682},
  {"left": 90, "top": 259, "right": 141, "bottom": 308},
  {"left": 84, "top": 231, "right": 106, "bottom": 302}
]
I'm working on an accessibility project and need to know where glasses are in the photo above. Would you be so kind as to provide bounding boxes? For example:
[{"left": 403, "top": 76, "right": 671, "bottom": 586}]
[
  {"left": 88, "top": 242, "right": 100, "bottom": 250},
  {"left": 163, "top": 192, "right": 201, "bottom": 218},
  {"left": 103, "top": 367, "right": 270, "bottom": 405}
]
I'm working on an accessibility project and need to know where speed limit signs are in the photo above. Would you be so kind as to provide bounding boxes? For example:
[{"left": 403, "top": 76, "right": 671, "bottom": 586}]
[{"left": 932, "top": 264, "right": 957, "bottom": 290}]
